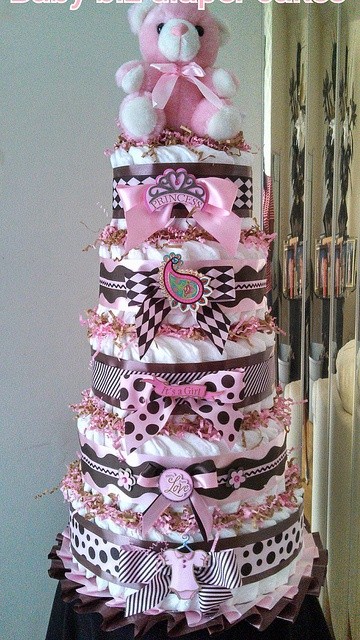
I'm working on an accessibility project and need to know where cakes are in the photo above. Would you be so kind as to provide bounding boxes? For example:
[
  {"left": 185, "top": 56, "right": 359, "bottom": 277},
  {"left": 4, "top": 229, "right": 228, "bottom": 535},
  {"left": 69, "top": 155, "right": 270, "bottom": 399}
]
[{"left": 33, "top": 129, "right": 329, "bottom": 638}]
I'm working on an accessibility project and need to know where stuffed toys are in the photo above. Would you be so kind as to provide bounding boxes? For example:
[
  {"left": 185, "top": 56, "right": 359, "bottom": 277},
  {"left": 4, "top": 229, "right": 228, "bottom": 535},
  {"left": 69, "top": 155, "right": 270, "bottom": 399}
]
[{"left": 119, "top": 7, "right": 237, "bottom": 139}]
